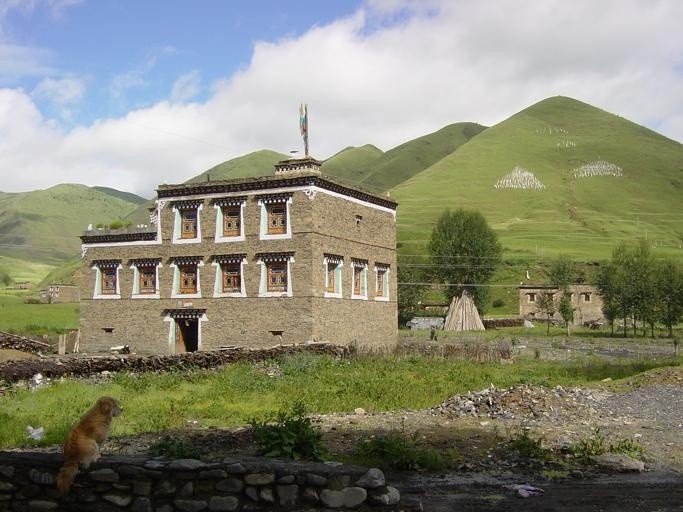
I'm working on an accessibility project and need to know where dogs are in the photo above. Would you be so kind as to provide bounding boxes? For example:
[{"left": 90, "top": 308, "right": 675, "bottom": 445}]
[{"left": 54, "top": 397, "right": 124, "bottom": 496}]
